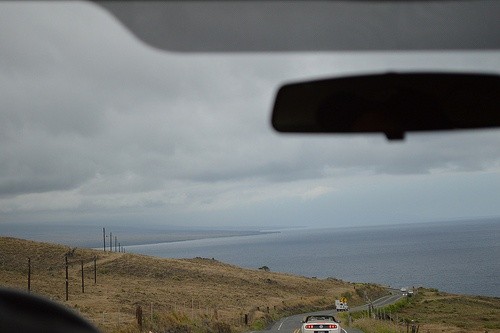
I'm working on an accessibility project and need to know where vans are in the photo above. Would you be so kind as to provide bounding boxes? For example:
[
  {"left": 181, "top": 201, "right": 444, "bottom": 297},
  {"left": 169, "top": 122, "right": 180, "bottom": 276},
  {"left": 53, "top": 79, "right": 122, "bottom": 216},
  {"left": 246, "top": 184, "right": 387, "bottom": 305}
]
[{"left": 336, "top": 302, "right": 348, "bottom": 311}]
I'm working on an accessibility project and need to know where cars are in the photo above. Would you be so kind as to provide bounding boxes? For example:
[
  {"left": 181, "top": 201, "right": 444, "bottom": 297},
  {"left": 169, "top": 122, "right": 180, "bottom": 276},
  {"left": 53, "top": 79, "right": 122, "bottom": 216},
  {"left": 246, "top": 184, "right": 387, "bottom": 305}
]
[{"left": 300, "top": 315, "right": 341, "bottom": 333}]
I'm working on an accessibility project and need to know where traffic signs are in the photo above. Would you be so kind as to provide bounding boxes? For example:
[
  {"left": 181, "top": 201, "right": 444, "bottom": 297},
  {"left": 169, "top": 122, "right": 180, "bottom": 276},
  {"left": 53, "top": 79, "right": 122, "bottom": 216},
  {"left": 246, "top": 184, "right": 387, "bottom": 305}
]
[{"left": 339, "top": 297, "right": 347, "bottom": 312}]
